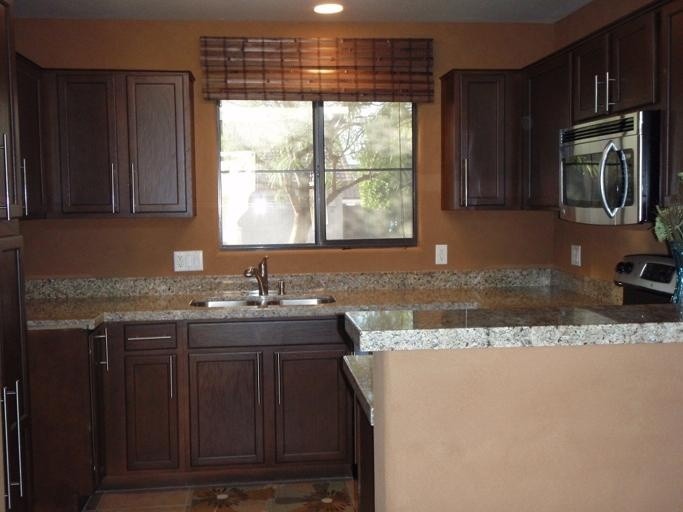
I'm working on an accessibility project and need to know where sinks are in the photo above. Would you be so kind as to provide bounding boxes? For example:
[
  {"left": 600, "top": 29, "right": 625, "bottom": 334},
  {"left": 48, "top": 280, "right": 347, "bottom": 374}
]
[
  {"left": 188, "top": 294, "right": 263, "bottom": 307},
  {"left": 262, "top": 293, "right": 338, "bottom": 307}
]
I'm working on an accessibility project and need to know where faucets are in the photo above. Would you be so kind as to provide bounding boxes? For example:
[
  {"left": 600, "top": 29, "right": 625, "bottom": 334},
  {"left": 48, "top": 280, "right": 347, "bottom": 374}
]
[{"left": 243, "top": 255, "right": 269, "bottom": 295}]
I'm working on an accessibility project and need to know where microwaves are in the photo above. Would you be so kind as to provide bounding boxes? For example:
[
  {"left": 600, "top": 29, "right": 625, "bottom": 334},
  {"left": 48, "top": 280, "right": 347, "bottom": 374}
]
[{"left": 556, "top": 108, "right": 658, "bottom": 230}]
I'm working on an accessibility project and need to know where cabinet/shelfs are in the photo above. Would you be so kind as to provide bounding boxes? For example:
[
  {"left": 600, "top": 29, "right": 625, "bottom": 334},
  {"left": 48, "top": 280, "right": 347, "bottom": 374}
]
[
  {"left": 0, "top": 0, "right": 32, "bottom": 511},
  {"left": 17, "top": 50, "right": 197, "bottom": 221}
]
